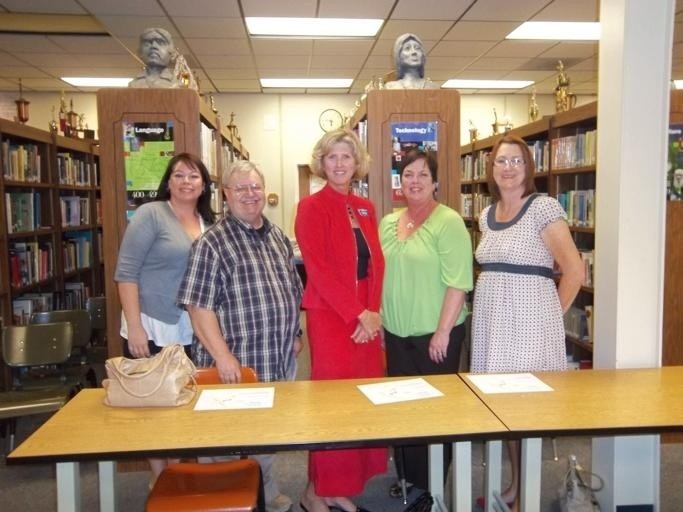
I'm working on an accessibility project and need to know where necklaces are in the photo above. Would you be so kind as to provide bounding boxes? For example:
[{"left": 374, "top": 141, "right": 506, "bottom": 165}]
[{"left": 405, "top": 198, "right": 434, "bottom": 229}]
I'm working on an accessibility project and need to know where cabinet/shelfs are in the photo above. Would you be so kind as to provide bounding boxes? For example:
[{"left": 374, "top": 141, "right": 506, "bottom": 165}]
[
  {"left": 0, "top": 117, "right": 106, "bottom": 438},
  {"left": 96, "top": 87, "right": 250, "bottom": 474},
  {"left": 344, "top": 88, "right": 462, "bottom": 229},
  {"left": 460, "top": 89, "right": 683, "bottom": 444}
]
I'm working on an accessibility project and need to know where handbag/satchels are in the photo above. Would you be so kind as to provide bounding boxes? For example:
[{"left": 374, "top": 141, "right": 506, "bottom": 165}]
[
  {"left": 558, "top": 455, "right": 605, "bottom": 512},
  {"left": 103, "top": 344, "right": 197, "bottom": 407}
]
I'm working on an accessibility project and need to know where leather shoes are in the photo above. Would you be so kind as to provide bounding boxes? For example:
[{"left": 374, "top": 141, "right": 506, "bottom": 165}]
[{"left": 264, "top": 479, "right": 513, "bottom": 512}]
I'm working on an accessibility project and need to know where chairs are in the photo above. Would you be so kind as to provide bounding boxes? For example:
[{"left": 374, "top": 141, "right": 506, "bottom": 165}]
[
  {"left": 144, "top": 366, "right": 265, "bottom": 512},
  {"left": 0, "top": 294, "right": 108, "bottom": 453}
]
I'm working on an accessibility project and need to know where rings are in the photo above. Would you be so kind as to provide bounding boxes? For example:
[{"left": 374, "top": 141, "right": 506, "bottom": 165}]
[{"left": 436, "top": 352, "right": 442, "bottom": 356}]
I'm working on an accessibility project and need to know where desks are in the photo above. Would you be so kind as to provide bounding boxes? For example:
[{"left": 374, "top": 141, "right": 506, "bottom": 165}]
[
  {"left": 456, "top": 365, "right": 683, "bottom": 512},
  {"left": 7, "top": 373, "right": 510, "bottom": 512}
]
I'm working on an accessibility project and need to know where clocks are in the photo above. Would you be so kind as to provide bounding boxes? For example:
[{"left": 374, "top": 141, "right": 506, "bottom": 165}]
[{"left": 319, "top": 109, "right": 343, "bottom": 133}]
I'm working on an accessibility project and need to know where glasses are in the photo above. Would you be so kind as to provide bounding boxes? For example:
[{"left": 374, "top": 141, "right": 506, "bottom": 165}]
[
  {"left": 492, "top": 158, "right": 524, "bottom": 168},
  {"left": 225, "top": 184, "right": 262, "bottom": 194}
]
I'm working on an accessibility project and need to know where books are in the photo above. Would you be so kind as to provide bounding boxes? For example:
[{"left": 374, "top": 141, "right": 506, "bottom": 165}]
[
  {"left": 458, "top": 150, "right": 494, "bottom": 305},
  {"left": 524, "top": 129, "right": 597, "bottom": 346},
  {"left": 2, "top": 136, "right": 104, "bottom": 323},
  {"left": 353, "top": 119, "right": 368, "bottom": 203},
  {"left": 200, "top": 122, "right": 242, "bottom": 232}
]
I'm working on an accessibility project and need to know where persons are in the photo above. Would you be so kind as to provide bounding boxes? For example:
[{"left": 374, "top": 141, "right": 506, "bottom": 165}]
[
  {"left": 172, "top": 161, "right": 304, "bottom": 511},
  {"left": 384, "top": 33, "right": 439, "bottom": 89},
  {"left": 292, "top": 129, "right": 386, "bottom": 512},
  {"left": 113, "top": 153, "right": 221, "bottom": 493},
  {"left": 374, "top": 149, "right": 472, "bottom": 498},
  {"left": 128, "top": 26, "right": 181, "bottom": 88},
  {"left": 470, "top": 137, "right": 585, "bottom": 512}
]
